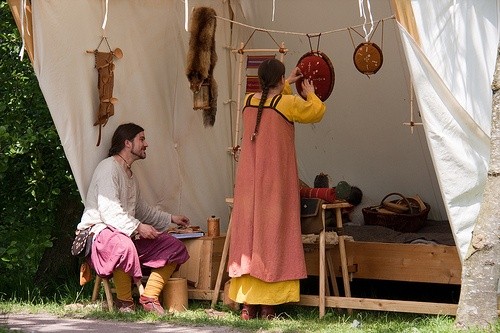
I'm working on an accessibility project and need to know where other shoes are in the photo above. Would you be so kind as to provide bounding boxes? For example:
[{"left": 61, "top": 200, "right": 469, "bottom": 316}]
[
  {"left": 137, "top": 295, "right": 165, "bottom": 315},
  {"left": 240, "top": 304, "right": 257, "bottom": 320},
  {"left": 115, "top": 298, "right": 134, "bottom": 313},
  {"left": 260, "top": 305, "right": 275, "bottom": 320}
]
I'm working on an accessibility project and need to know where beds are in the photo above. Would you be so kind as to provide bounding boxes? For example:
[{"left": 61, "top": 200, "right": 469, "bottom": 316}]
[{"left": 223, "top": 218, "right": 461, "bottom": 316}]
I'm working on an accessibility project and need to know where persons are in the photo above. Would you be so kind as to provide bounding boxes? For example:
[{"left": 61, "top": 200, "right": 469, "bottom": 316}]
[
  {"left": 226, "top": 58, "right": 327, "bottom": 320},
  {"left": 75, "top": 123, "right": 190, "bottom": 316}
]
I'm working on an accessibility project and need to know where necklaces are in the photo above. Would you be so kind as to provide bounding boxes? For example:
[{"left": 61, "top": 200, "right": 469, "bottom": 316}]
[{"left": 116, "top": 152, "right": 131, "bottom": 169}]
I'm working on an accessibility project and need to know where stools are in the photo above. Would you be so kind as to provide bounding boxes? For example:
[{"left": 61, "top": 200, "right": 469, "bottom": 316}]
[{"left": 92, "top": 274, "right": 118, "bottom": 314}]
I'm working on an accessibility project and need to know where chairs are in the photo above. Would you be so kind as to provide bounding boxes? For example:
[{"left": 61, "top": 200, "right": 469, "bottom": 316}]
[{"left": 211, "top": 188, "right": 356, "bottom": 318}]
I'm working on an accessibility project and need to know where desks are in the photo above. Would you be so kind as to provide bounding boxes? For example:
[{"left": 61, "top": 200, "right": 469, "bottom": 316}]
[{"left": 169, "top": 229, "right": 228, "bottom": 303}]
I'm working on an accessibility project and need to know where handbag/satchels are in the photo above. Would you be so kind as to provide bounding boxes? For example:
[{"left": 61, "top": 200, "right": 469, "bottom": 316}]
[
  {"left": 71, "top": 232, "right": 92, "bottom": 258},
  {"left": 300, "top": 198, "right": 336, "bottom": 234}
]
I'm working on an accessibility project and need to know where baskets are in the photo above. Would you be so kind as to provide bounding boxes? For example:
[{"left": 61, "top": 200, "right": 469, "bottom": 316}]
[{"left": 361, "top": 193, "right": 431, "bottom": 233}]
